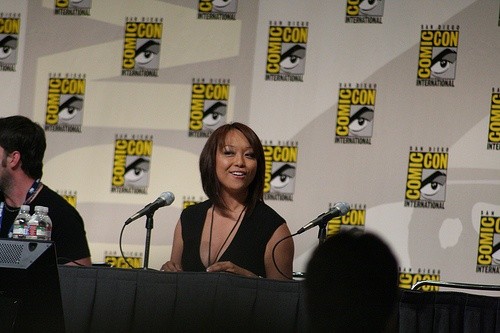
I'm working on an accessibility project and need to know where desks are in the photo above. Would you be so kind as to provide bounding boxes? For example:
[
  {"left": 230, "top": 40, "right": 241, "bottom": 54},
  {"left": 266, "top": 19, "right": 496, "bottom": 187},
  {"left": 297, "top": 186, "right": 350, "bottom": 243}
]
[{"left": 58, "top": 265, "right": 500, "bottom": 333}]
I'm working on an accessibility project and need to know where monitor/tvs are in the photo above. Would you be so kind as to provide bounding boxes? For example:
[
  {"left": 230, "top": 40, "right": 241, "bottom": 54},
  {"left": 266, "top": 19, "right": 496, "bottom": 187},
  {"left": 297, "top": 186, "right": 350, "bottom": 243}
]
[{"left": 0, "top": 237, "right": 64, "bottom": 332}]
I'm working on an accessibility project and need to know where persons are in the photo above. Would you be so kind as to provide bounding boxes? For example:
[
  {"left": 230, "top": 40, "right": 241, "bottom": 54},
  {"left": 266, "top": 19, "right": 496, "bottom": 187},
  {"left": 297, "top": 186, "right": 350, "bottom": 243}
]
[
  {"left": 0, "top": 115, "right": 91, "bottom": 267},
  {"left": 161, "top": 122, "right": 294, "bottom": 281},
  {"left": 304, "top": 228, "right": 399, "bottom": 333}
]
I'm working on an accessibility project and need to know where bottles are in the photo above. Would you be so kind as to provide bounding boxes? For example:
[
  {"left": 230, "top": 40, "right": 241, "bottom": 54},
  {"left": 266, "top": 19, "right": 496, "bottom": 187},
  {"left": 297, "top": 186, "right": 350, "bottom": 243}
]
[
  {"left": 25, "top": 206, "right": 48, "bottom": 240},
  {"left": 12, "top": 205, "right": 31, "bottom": 239},
  {"left": 43, "top": 207, "right": 53, "bottom": 241}
]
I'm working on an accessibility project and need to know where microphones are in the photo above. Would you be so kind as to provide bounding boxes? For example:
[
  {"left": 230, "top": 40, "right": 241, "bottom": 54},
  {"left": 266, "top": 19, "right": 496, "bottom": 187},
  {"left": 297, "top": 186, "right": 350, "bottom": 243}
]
[
  {"left": 124, "top": 191, "right": 175, "bottom": 225},
  {"left": 297, "top": 201, "right": 350, "bottom": 234}
]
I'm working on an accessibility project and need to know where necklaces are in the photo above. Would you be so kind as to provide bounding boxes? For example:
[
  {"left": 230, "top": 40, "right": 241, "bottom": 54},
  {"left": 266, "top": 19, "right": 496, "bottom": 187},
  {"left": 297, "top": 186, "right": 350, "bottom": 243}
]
[
  {"left": 208, "top": 203, "right": 247, "bottom": 267},
  {"left": 4, "top": 182, "right": 42, "bottom": 213}
]
